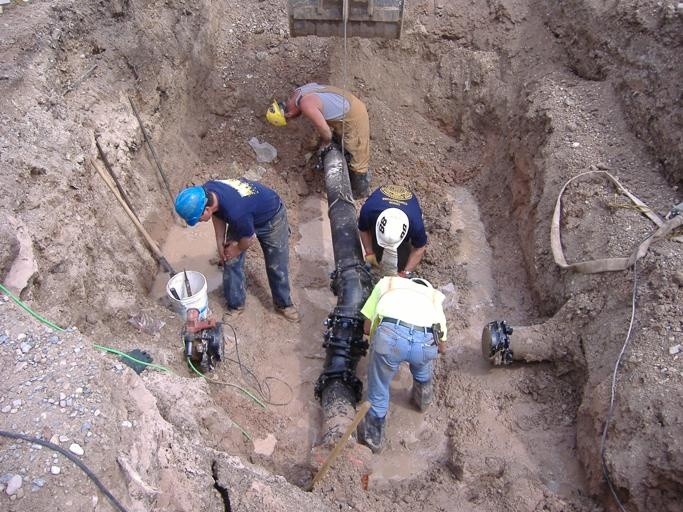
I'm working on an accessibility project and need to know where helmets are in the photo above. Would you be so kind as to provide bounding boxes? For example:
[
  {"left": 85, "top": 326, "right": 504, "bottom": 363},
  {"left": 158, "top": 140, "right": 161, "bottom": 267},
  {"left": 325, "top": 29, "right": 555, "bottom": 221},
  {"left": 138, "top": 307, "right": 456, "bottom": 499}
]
[
  {"left": 265, "top": 97, "right": 288, "bottom": 126},
  {"left": 410, "top": 278, "right": 433, "bottom": 288},
  {"left": 174, "top": 185, "right": 208, "bottom": 226},
  {"left": 375, "top": 207, "right": 409, "bottom": 250}
]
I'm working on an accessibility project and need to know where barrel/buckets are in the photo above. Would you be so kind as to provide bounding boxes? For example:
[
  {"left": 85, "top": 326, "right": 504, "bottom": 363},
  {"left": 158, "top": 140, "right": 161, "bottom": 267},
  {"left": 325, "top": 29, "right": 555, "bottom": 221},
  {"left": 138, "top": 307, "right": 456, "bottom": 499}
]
[{"left": 166, "top": 270, "right": 209, "bottom": 322}]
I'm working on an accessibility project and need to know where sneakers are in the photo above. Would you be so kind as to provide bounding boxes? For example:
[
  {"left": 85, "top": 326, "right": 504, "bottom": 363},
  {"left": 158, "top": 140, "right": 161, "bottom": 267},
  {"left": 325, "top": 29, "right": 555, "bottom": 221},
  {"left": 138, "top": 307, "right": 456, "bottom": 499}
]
[
  {"left": 274, "top": 304, "right": 300, "bottom": 322},
  {"left": 222, "top": 302, "right": 245, "bottom": 322}
]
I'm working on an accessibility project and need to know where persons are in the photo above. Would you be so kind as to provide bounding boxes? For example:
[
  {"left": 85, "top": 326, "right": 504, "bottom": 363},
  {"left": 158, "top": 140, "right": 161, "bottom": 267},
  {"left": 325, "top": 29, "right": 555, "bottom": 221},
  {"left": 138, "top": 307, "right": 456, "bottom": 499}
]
[
  {"left": 264, "top": 81, "right": 372, "bottom": 199},
  {"left": 173, "top": 177, "right": 300, "bottom": 325},
  {"left": 356, "top": 273, "right": 448, "bottom": 455},
  {"left": 355, "top": 184, "right": 428, "bottom": 279}
]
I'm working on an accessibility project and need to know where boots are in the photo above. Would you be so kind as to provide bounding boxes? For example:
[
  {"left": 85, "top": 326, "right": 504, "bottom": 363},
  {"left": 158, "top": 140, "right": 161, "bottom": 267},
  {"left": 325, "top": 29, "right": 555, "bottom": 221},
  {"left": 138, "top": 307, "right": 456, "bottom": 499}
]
[
  {"left": 361, "top": 411, "right": 386, "bottom": 452},
  {"left": 349, "top": 169, "right": 370, "bottom": 200},
  {"left": 411, "top": 377, "right": 432, "bottom": 413}
]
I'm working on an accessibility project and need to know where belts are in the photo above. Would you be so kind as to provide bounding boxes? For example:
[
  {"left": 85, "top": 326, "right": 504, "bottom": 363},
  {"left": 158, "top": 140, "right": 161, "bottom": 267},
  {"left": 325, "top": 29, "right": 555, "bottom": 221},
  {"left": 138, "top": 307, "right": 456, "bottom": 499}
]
[{"left": 381, "top": 316, "right": 434, "bottom": 334}]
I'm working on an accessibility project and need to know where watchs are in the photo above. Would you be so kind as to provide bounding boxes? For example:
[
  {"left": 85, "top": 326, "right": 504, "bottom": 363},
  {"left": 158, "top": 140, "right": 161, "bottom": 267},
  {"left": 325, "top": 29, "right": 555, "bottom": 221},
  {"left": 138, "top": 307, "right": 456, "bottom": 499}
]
[{"left": 402, "top": 269, "right": 412, "bottom": 278}]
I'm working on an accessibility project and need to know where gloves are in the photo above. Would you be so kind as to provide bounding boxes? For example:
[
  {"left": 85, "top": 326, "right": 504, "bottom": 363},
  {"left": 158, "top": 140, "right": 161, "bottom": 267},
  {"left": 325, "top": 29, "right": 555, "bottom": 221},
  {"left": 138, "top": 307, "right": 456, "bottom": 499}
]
[
  {"left": 119, "top": 349, "right": 154, "bottom": 375},
  {"left": 364, "top": 253, "right": 380, "bottom": 269}
]
